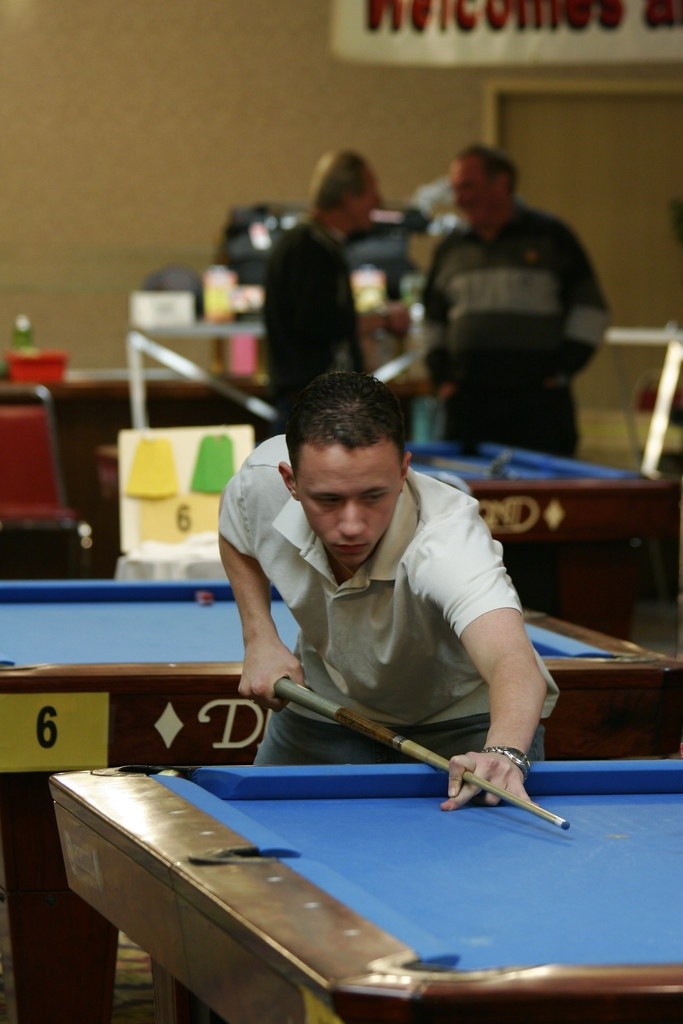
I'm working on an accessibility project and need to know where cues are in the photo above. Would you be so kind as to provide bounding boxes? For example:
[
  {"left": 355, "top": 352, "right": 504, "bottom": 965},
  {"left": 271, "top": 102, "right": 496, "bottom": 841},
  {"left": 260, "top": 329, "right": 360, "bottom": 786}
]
[{"left": 271, "top": 675, "right": 574, "bottom": 833}]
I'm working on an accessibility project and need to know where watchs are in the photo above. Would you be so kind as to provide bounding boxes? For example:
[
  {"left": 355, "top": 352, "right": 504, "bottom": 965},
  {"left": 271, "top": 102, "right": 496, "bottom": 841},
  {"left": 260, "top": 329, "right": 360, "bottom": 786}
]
[{"left": 482, "top": 746, "right": 530, "bottom": 784}]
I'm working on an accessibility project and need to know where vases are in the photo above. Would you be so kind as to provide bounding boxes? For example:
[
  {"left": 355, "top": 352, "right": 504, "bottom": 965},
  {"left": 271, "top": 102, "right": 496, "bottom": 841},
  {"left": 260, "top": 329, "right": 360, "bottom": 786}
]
[{"left": 6, "top": 349, "right": 69, "bottom": 384}]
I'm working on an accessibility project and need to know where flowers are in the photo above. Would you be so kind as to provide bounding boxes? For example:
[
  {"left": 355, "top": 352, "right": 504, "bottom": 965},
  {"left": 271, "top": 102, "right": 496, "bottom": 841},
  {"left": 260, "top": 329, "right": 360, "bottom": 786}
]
[{"left": 9, "top": 315, "right": 39, "bottom": 355}]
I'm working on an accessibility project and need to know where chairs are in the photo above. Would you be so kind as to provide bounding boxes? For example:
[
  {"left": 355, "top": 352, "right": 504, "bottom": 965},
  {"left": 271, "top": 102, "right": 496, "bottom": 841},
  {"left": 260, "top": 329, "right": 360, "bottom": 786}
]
[{"left": 0, "top": 383, "right": 93, "bottom": 579}]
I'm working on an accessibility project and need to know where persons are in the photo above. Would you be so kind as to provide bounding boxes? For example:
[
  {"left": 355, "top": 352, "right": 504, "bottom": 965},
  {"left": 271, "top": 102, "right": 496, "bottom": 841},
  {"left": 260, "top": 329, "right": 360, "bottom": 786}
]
[
  {"left": 218, "top": 370, "right": 560, "bottom": 811},
  {"left": 261, "top": 147, "right": 610, "bottom": 459}
]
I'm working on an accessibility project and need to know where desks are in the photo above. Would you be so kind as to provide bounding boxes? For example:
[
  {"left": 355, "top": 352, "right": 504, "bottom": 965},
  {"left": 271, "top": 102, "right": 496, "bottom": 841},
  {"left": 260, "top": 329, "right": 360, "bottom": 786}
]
[
  {"left": 48, "top": 760, "right": 683, "bottom": 1024},
  {"left": 405, "top": 442, "right": 683, "bottom": 642},
  {"left": 0, "top": 577, "right": 683, "bottom": 1024}
]
